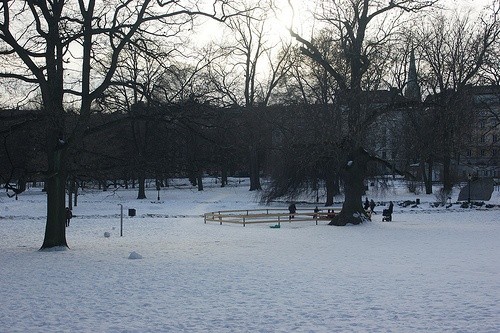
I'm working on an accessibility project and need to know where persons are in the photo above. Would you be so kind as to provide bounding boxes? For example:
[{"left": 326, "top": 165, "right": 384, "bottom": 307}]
[
  {"left": 326, "top": 209, "right": 335, "bottom": 218},
  {"left": 364, "top": 198, "right": 379, "bottom": 216},
  {"left": 65, "top": 205, "right": 73, "bottom": 227},
  {"left": 387, "top": 198, "right": 395, "bottom": 221},
  {"left": 288, "top": 203, "right": 297, "bottom": 222},
  {"left": 312, "top": 206, "right": 321, "bottom": 220},
  {"left": 410, "top": 201, "right": 418, "bottom": 209}
]
